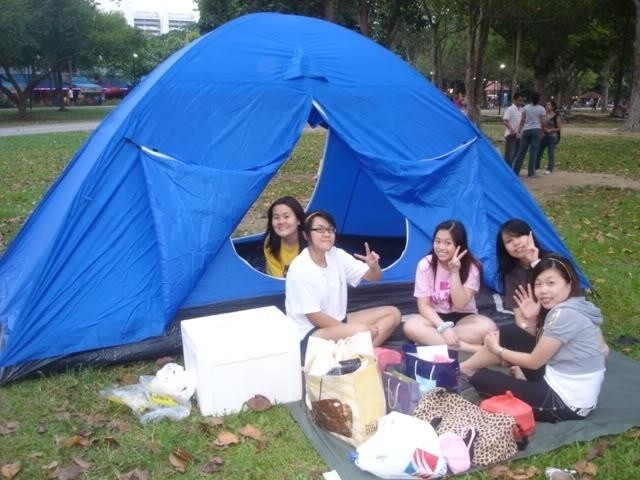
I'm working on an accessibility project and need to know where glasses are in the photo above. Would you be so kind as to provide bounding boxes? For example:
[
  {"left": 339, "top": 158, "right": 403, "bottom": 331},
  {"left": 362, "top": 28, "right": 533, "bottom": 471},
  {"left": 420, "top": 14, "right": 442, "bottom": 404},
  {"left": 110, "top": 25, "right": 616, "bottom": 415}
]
[{"left": 312, "top": 225, "right": 336, "bottom": 233}]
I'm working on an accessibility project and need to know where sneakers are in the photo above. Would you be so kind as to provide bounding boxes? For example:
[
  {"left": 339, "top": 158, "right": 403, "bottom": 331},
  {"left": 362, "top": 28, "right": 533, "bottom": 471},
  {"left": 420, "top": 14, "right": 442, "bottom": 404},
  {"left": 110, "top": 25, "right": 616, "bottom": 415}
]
[{"left": 528, "top": 169, "right": 551, "bottom": 178}]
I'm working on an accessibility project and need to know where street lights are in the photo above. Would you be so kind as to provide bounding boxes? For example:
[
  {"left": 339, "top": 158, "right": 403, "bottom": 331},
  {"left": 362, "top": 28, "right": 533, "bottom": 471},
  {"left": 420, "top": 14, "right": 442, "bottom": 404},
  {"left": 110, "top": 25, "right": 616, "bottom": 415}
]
[
  {"left": 132, "top": 50, "right": 138, "bottom": 84},
  {"left": 429, "top": 62, "right": 520, "bottom": 114}
]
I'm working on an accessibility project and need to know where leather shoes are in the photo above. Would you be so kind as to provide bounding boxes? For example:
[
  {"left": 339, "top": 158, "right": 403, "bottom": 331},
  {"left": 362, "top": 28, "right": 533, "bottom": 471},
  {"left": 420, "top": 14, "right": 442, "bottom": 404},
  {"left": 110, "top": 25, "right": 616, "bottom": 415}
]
[{"left": 544, "top": 467, "right": 577, "bottom": 480}]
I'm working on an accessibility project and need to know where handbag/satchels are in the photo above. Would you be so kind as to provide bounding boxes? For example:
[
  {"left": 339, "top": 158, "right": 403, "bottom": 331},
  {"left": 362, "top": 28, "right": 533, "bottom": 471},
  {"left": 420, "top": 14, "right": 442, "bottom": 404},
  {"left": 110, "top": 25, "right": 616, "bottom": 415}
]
[{"left": 304, "top": 331, "right": 536, "bottom": 480}]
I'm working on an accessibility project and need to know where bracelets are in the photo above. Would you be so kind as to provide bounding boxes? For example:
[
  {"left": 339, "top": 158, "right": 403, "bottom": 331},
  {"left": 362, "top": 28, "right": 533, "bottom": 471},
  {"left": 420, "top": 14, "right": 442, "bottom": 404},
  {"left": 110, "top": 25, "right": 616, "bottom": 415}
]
[
  {"left": 522, "top": 322, "right": 539, "bottom": 329},
  {"left": 496, "top": 347, "right": 504, "bottom": 359},
  {"left": 438, "top": 321, "right": 454, "bottom": 333}
]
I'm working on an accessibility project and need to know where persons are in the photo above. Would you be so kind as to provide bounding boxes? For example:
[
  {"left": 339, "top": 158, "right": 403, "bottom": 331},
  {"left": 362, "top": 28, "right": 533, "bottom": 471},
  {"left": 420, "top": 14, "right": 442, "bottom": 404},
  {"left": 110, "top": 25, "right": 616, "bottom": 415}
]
[
  {"left": 63, "top": 87, "right": 106, "bottom": 105},
  {"left": 284, "top": 209, "right": 402, "bottom": 366},
  {"left": 460, "top": 253, "right": 609, "bottom": 425},
  {"left": 502, "top": 92, "right": 602, "bottom": 180},
  {"left": 495, "top": 219, "right": 552, "bottom": 330},
  {"left": 262, "top": 197, "right": 307, "bottom": 280},
  {"left": 403, "top": 220, "right": 498, "bottom": 353}
]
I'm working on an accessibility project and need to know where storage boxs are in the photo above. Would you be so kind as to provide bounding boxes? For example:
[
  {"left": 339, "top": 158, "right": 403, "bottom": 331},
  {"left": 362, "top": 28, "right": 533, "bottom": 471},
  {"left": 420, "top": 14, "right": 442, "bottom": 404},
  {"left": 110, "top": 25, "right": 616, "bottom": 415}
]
[{"left": 180, "top": 304, "right": 303, "bottom": 417}]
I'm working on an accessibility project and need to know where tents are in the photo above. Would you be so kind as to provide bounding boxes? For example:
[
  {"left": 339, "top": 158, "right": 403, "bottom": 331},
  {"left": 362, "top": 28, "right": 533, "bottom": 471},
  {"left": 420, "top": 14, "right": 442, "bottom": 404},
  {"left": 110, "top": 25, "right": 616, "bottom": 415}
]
[{"left": 0, "top": 10, "right": 603, "bottom": 386}]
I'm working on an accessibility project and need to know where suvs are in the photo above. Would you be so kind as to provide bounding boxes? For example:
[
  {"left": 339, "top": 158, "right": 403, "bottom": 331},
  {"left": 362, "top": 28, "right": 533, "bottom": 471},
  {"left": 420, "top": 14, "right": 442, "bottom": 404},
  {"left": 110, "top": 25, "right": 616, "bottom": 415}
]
[{"left": 0, "top": 74, "right": 131, "bottom": 106}]
